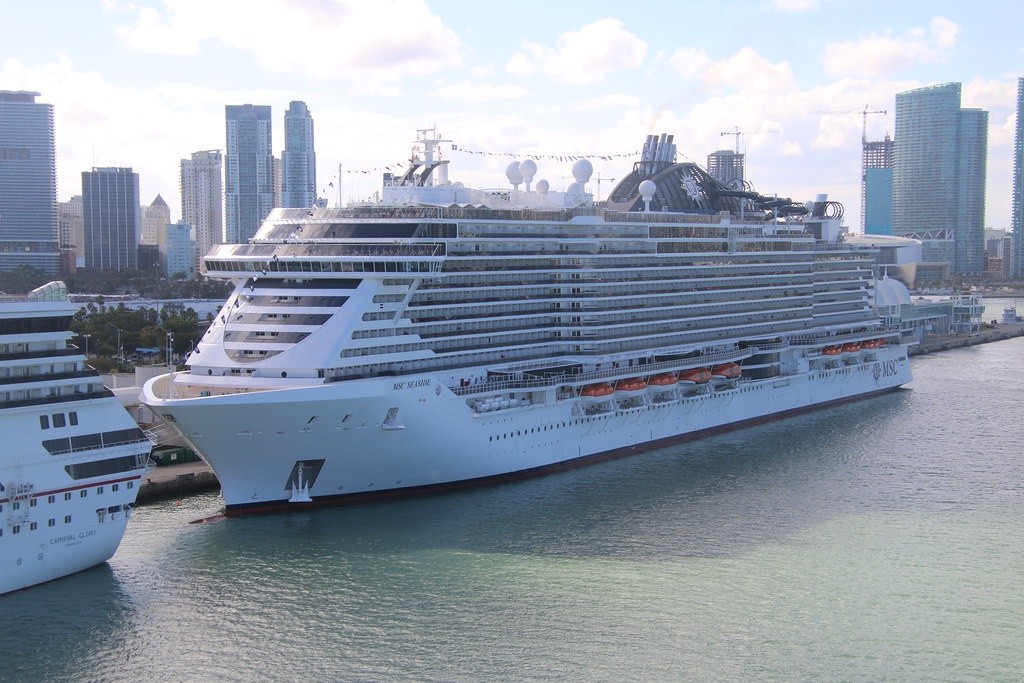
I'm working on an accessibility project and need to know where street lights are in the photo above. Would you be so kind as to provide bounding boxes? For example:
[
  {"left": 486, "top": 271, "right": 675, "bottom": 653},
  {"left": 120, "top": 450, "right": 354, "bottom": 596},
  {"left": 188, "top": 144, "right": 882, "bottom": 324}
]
[
  {"left": 152, "top": 261, "right": 159, "bottom": 315},
  {"left": 83, "top": 334, "right": 92, "bottom": 358},
  {"left": 116, "top": 328, "right": 123, "bottom": 367},
  {"left": 165, "top": 332, "right": 170, "bottom": 366},
  {"left": 189, "top": 340, "right": 194, "bottom": 351}
]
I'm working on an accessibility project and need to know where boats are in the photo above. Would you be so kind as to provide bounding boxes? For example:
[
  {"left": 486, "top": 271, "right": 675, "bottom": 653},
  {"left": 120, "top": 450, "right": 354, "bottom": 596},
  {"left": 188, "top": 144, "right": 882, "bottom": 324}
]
[
  {"left": 1002, "top": 307, "right": 1024, "bottom": 323},
  {"left": 0, "top": 299, "right": 141, "bottom": 596}
]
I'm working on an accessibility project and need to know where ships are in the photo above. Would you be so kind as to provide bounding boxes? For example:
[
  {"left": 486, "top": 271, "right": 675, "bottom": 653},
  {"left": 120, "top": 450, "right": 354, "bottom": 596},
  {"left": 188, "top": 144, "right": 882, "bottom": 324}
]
[{"left": 133, "top": 132, "right": 915, "bottom": 514}]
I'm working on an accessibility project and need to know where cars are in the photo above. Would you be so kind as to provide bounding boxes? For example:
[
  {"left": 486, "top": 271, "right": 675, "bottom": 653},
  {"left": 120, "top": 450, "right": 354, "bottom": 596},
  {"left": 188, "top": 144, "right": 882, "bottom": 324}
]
[{"left": 111, "top": 351, "right": 142, "bottom": 365}]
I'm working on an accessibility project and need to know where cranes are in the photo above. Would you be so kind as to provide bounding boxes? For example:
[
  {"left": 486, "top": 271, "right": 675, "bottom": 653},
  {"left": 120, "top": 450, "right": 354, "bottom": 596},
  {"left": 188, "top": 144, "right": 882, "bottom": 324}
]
[
  {"left": 802, "top": 102, "right": 888, "bottom": 142},
  {"left": 718, "top": 126, "right": 779, "bottom": 153}
]
